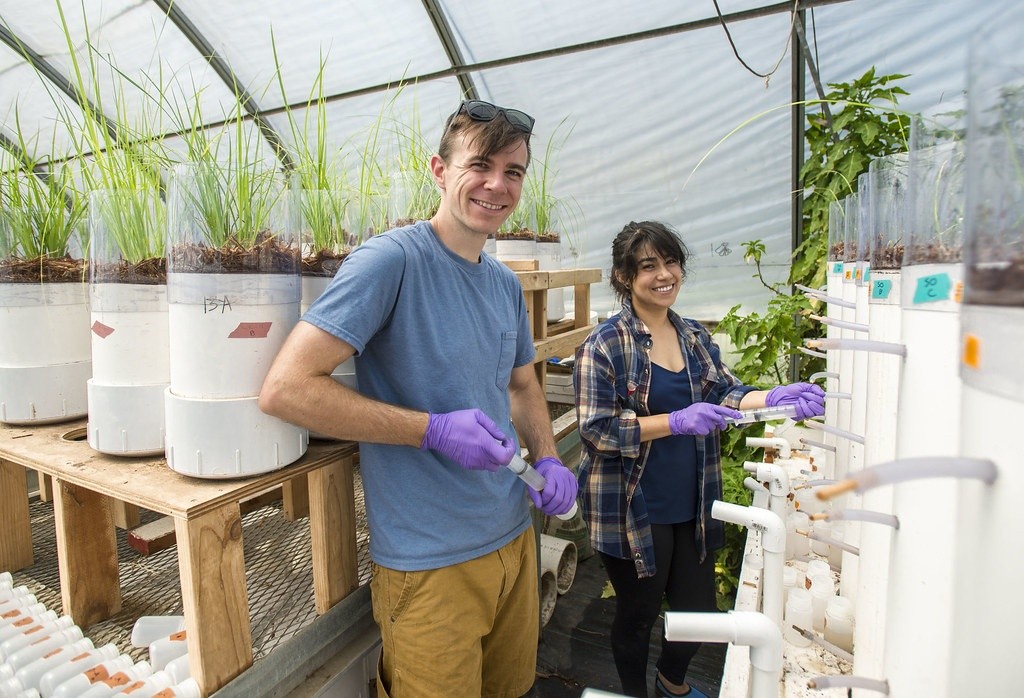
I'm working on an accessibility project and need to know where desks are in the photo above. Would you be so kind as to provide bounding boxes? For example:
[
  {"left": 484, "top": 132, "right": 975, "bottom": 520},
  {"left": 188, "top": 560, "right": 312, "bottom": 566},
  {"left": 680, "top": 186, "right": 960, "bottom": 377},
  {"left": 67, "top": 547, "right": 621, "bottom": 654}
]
[{"left": 2, "top": 263, "right": 603, "bottom": 698}]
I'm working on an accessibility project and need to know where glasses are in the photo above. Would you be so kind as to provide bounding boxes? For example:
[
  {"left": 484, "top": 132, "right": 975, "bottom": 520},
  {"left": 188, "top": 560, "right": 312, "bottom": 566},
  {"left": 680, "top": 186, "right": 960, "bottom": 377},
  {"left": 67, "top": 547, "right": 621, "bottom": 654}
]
[{"left": 439, "top": 99, "right": 535, "bottom": 145}]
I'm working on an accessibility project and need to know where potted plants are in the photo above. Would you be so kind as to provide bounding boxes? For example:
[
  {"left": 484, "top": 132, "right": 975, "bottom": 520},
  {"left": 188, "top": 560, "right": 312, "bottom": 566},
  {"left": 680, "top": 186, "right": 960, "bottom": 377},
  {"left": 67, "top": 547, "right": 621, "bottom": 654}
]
[
  {"left": 713, "top": 3, "right": 1023, "bottom": 698},
  {"left": 0, "top": 92, "right": 583, "bottom": 480}
]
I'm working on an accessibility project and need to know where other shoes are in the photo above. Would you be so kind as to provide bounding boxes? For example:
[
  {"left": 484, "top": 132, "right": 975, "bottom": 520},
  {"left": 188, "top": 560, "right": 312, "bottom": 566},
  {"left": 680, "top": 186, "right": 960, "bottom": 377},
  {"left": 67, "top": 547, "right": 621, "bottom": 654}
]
[{"left": 656, "top": 674, "right": 709, "bottom": 698}]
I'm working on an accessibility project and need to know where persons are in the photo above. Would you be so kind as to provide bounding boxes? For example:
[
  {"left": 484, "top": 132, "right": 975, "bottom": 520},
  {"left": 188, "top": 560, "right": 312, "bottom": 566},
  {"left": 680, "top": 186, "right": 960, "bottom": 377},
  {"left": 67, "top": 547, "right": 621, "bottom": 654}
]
[
  {"left": 256, "top": 98, "right": 579, "bottom": 697},
  {"left": 572, "top": 220, "right": 826, "bottom": 698}
]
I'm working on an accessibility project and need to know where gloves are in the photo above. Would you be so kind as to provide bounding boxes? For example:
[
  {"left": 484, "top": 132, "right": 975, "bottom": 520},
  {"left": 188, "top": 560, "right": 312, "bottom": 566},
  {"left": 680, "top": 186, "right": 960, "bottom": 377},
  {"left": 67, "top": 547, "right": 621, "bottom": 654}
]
[
  {"left": 670, "top": 403, "right": 742, "bottom": 436},
  {"left": 421, "top": 407, "right": 515, "bottom": 472},
  {"left": 530, "top": 457, "right": 580, "bottom": 516},
  {"left": 767, "top": 383, "right": 826, "bottom": 420}
]
